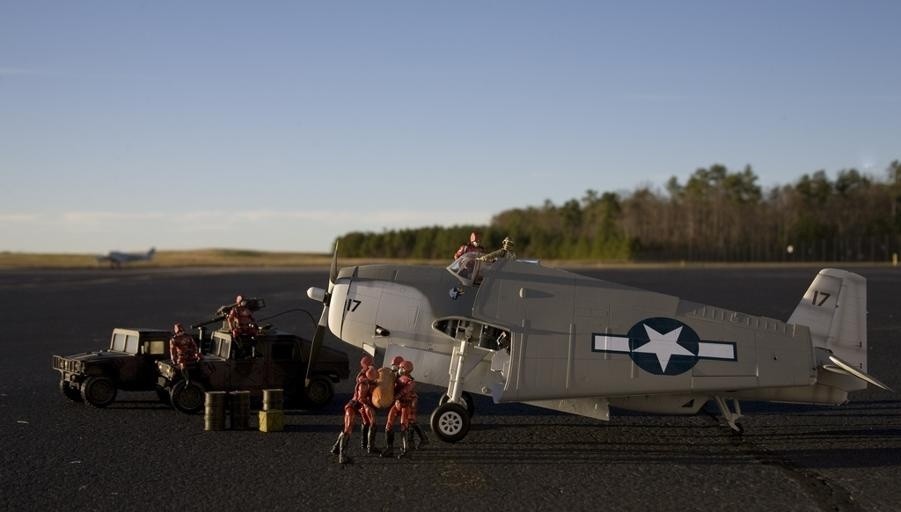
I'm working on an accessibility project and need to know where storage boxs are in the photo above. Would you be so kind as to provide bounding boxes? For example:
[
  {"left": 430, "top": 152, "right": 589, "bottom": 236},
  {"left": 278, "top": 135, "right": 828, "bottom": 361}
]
[{"left": 258, "top": 409, "right": 284, "bottom": 432}]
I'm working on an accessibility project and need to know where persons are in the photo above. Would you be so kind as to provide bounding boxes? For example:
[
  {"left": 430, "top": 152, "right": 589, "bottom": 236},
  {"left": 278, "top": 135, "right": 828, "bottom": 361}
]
[
  {"left": 228, "top": 295, "right": 258, "bottom": 352},
  {"left": 170, "top": 322, "right": 202, "bottom": 389},
  {"left": 453, "top": 231, "right": 517, "bottom": 283},
  {"left": 331, "top": 356, "right": 429, "bottom": 463}
]
